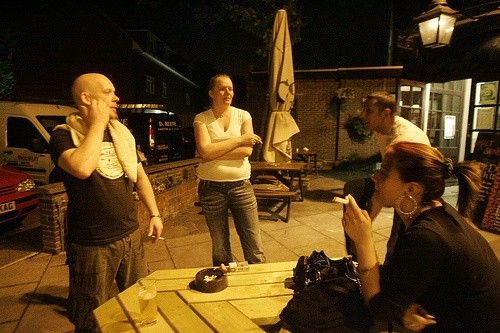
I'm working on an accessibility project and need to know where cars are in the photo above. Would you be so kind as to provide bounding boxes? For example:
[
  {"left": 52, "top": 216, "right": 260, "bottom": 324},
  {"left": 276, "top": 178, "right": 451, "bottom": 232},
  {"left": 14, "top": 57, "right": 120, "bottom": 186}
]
[{"left": 0, "top": 164, "right": 40, "bottom": 227}]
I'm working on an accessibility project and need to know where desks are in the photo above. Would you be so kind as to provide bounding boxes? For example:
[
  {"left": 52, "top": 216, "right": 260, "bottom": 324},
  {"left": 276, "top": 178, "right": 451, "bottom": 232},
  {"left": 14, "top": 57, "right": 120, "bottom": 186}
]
[
  {"left": 92, "top": 257, "right": 358, "bottom": 333},
  {"left": 292, "top": 149, "right": 320, "bottom": 176},
  {"left": 249, "top": 161, "right": 308, "bottom": 223}
]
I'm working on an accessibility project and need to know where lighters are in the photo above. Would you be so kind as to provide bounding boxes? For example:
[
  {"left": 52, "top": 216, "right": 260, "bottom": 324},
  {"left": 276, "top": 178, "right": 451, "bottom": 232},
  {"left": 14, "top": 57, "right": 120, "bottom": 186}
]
[{"left": 221, "top": 263, "right": 228, "bottom": 273}]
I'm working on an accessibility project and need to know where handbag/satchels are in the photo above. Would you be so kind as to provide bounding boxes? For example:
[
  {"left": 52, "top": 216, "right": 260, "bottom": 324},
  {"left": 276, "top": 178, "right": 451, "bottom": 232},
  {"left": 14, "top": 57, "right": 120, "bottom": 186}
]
[{"left": 293, "top": 251, "right": 357, "bottom": 294}]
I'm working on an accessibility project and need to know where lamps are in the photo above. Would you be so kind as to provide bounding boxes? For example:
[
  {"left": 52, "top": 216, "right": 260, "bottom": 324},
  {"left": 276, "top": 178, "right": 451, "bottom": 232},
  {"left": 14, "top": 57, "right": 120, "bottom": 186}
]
[{"left": 413, "top": 0, "right": 463, "bottom": 49}]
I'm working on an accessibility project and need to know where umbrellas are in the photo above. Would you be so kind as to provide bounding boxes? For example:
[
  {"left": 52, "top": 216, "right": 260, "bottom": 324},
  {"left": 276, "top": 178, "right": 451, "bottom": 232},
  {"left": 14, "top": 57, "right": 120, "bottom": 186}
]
[{"left": 262, "top": 4, "right": 301, "bottom": 165}]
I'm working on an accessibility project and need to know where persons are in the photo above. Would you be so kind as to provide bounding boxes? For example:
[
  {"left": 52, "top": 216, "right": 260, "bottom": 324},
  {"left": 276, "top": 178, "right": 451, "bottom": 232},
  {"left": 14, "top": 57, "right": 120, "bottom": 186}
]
[
  {"left": 342, "top": 142, "right": 500, "bottom": 333},
  {"left": 193, "top": 74, "right": 266, "bottom": 267},
  {"left": 342, "top": 91, "right": 431, "bottom": 263},
  {"left": 50, "top": 73, "right": 163, "bottom": 333}
]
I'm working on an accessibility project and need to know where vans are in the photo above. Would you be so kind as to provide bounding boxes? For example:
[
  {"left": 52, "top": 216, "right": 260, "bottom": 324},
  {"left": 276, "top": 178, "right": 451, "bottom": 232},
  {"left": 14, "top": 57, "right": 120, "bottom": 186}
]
[
  {"left": 116, "top": 107, "right": 184, "bottom": 165},
  {"left": 0, "top": 100, "right": 81, "bottom": 185}
]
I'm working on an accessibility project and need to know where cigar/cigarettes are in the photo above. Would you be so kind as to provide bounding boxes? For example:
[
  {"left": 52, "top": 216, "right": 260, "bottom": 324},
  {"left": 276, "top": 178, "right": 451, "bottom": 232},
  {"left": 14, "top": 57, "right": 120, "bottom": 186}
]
[
  {"left": 333, "top": 197, "right": 351, "bottom": 206},
  {"left": 258, "top": 140, "right": 263, "bottom": 144},
  {"left": 151, "top": 236, "right": 163, "bottom": 240}
]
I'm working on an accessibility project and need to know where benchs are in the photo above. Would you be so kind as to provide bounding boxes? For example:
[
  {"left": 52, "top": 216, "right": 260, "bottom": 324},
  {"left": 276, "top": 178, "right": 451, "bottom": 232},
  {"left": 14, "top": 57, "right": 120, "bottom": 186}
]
[
  {"left": 253, "top": 189, "right": 298, "bottom": 223},
  {"left": 256, "top": 176, "right": 311, "bottom": 189}
]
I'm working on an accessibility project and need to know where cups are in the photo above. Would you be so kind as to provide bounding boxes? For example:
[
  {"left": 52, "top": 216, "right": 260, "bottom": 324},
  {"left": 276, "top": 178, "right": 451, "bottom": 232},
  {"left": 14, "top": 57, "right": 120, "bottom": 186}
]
[{"left": 135, "top": 277, "right": 158, "bottom": 326}]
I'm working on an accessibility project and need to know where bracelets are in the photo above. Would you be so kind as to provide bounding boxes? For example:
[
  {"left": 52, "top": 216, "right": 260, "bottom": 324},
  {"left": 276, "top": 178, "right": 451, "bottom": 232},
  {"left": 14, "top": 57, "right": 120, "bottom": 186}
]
[{"left": 150, "top": 215, "right": 162, "bottom": 220}]
